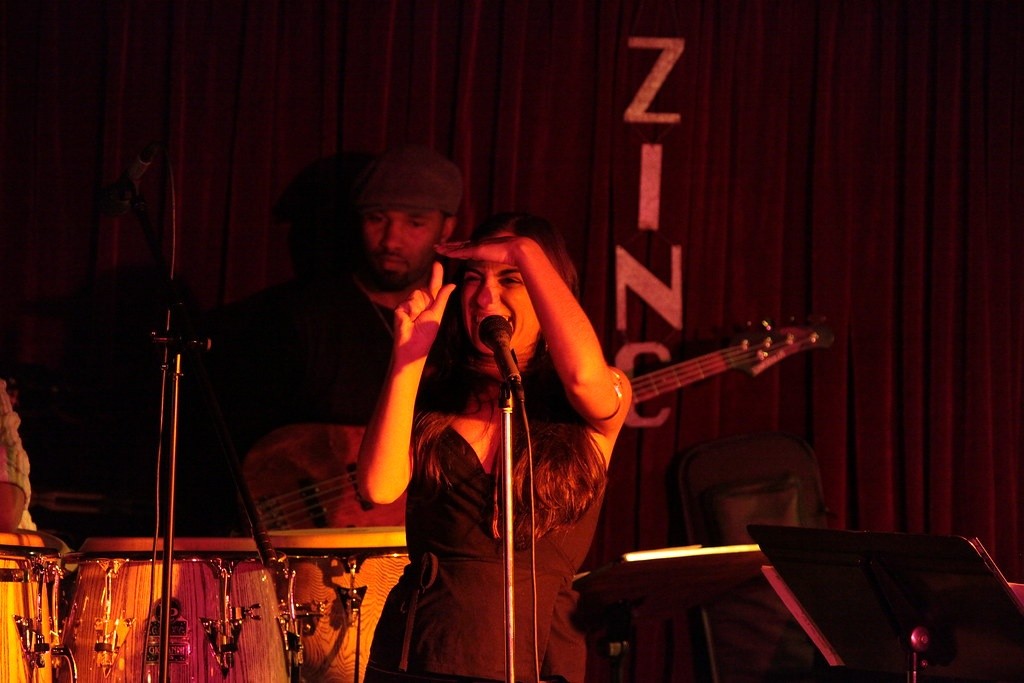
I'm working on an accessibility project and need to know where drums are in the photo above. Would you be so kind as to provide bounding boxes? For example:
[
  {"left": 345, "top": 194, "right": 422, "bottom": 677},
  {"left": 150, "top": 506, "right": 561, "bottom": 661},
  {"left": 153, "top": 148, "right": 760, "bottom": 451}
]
[
  {"left": 49, "top": 534, "right": 308, "bottom": 683},
  {"left": 0, "top": 524, "right": 76, "bottom": 683},
  {"left": 258, "top": 524, "right": 413, "bottom": 683}
]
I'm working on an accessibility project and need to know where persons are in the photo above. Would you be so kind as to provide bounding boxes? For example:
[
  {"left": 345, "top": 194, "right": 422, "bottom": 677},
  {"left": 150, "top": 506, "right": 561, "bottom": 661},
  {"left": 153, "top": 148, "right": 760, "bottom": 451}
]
[
  {"left": 0, "top": 380, "right": 38, "bottom": 533},
  {"left": 356, "top": 210, "right": 632, "bottom": 683},
  {"left": 177, "top": 144, "right": 474, "bottom": 537}
]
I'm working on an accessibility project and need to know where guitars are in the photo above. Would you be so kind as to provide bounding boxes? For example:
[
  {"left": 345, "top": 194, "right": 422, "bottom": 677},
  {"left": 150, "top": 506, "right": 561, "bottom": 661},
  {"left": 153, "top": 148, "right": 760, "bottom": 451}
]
[{"left": 231, "top": 311, "right": 832, "bottom": 543}]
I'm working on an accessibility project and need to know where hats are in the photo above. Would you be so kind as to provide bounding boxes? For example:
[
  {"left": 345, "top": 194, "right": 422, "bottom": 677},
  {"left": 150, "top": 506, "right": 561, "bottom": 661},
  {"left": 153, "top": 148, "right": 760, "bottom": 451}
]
[{"left": 357, "top": 146, "right": 464, "bottom": 217}]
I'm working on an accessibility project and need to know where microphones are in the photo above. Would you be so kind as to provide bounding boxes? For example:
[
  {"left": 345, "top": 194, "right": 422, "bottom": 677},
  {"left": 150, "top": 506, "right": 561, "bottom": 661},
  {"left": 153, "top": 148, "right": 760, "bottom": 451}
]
[
  {"left": 100, "top": 142, "right": 158, "bottom": 218},
  {"left": 478, "top": 314, "right": 524, "bottom": 402}
]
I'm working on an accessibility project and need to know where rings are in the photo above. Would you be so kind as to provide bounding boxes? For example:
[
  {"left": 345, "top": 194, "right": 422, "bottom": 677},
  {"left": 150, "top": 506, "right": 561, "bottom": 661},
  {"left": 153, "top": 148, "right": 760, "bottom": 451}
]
[{"left": 460, "top": 240, "right": 471, "bottom": 249}]
